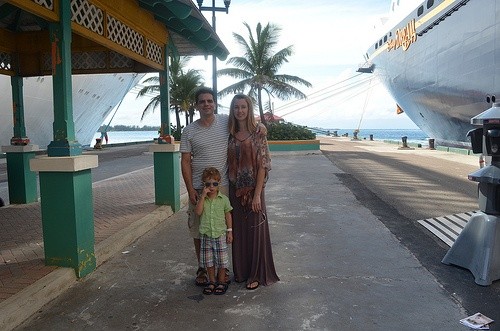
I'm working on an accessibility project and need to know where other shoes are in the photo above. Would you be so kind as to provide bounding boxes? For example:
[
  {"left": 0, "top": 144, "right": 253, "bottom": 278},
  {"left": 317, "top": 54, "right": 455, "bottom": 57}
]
[{"left": 246, "top": 280, "right": 259, "bottom": 290}]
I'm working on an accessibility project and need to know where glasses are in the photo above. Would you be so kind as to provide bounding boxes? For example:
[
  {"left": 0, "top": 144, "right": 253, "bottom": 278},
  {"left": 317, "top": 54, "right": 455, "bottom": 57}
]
[
  {"left": 246, "top": 208, "right": 266, "bottom": 227},
  {"left": 205, "top": 181, "right": 219, "bottom": 188},
  {"left": 197, "top": 99, "right": 213, "bottom": 105}
]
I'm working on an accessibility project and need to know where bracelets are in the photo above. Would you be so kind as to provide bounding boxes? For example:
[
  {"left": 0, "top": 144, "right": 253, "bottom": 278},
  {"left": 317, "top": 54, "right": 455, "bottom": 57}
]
[{"left": 227, "top": 227, "right": 232, "bottom": 231}]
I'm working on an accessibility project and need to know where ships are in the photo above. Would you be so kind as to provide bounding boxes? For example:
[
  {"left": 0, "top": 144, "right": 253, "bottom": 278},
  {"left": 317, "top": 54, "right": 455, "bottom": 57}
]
[{"left": 354, "top": 0, "right": 500, "bottom": 150}]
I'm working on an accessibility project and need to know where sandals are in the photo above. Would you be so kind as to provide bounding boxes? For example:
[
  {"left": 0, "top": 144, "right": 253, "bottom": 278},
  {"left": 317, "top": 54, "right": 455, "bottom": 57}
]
[
  {"left": 195, "top": 267, "right": 208, "bottom": 286},
  {"left": 203, "top": 281, "right": 216, "bottom": 293},
  {"left": 214, "top": 282, "right": 228, "bottom": 295},
  {"left": 223, "top": 269, "right": 232, "bottom": 284}
]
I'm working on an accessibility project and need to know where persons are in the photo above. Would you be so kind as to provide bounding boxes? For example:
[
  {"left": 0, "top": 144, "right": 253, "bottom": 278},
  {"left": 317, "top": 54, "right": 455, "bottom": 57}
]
[
  {"left": 227, "top": 94, "right": 281, "bottom": 290},
  {"left": 180, "top": 87, "right": 267, "bottom": 290},
  {"left": 101, "top": 124, "right": 108, "bottom": 144},
  {"left": 194, "top": 166, "right": 233, "bottom": 295}
]
